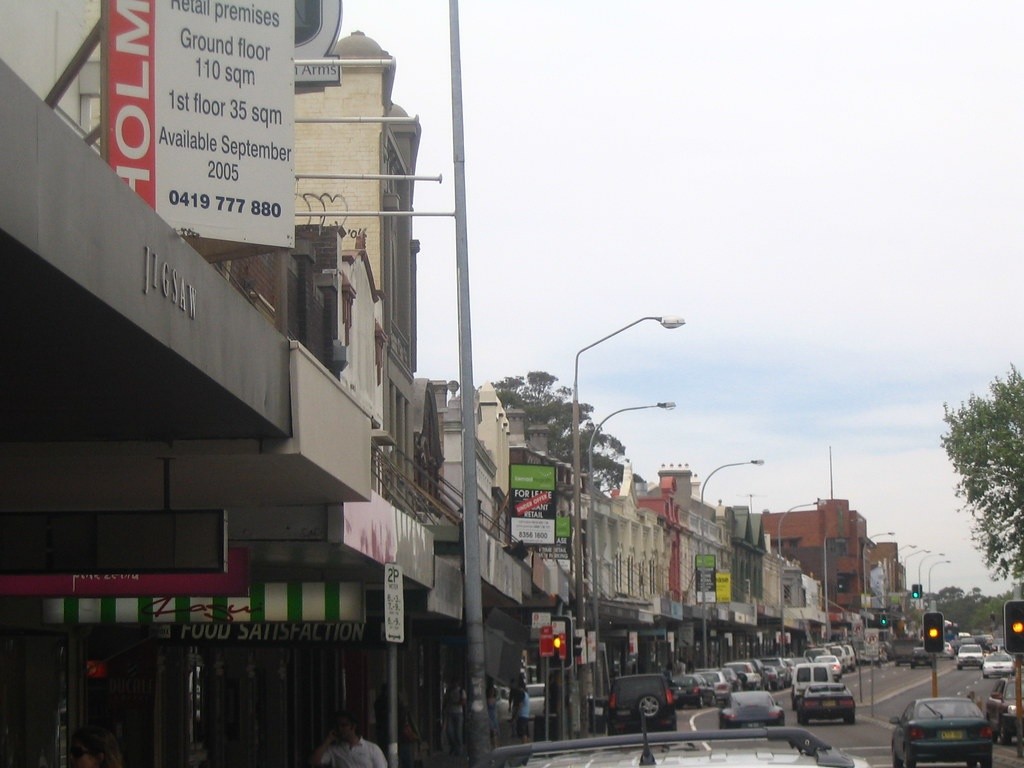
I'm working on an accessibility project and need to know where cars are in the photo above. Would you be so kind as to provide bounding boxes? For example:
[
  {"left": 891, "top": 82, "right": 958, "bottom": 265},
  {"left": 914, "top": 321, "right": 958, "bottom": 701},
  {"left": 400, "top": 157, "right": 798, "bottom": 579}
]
[
  {"left": 910, "top": 631, "right": 1016, "bottom": 680},
  {"left": 498, "top": 684, "right": 558, "bottom": 721},
  {"left": 986, "top": 677, "right": 1024, "bottom": 743},
  {"left": 671, "top": 673, "right": 718, "bottom": 708},
  {"left": 695, "top": 644, "right": 857, "bottom": 724},
  {"left": 719, "top": 692, "right": 785, "bottom": 731},
  {"left": 888, "top": 696, "right": 994, "bottom": 768}
]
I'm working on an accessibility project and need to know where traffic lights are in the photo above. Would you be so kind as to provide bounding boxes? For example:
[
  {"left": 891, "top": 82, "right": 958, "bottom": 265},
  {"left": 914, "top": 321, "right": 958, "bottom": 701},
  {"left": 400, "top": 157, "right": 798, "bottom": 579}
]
[
  {"left": 922, "top": 612, "right": 944, "bottom": 654},
  {"left": 911, "top": 584, "right": 922, "bottom": 599},
  {"left": 547, "top": 616, "right": 582, "bottom": 672},
  {"left": 880, "top": 613, "right": 885, "bottom": 625},
  {"left": 1004, "top": 599, "right": 1024, "bottom": 654}
]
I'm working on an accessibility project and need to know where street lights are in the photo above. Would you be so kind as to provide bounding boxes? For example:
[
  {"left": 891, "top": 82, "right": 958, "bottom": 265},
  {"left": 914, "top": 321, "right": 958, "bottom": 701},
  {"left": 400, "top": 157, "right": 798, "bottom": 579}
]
[
  {"left": 701, "top": 458, "right": 766, "bottom": 667},
  {"left": 778, "top": 499, "right": 827, "bottom": 655},
  {"left": 574, "top": 315, "right": 687, "bottom": 734},
  {"left": 862, "top": 532, "right": 895, "bottom": 628},
  {"left": 896, "top": 544, "right": 952, "bottom": 640}
]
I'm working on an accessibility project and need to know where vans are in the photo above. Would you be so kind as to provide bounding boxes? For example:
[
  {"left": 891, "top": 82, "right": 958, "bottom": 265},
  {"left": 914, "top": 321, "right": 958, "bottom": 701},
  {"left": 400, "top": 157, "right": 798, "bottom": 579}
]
[{"left": 791, "top": 662, "right": 834, "bottom": 710}]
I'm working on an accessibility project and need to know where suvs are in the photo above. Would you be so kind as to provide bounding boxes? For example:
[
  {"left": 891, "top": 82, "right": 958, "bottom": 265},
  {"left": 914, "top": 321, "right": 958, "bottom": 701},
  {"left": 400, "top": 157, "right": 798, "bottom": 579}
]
[{"left": 608, "top": 673, "right": 677, "bottom": 735}]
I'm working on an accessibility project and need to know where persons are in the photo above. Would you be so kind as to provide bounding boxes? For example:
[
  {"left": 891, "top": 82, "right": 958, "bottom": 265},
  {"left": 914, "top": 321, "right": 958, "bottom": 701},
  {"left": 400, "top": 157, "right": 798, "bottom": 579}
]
[
  {"left": 68, "top": 726, "right": 124, "bottom": 768},
  {"left": 371, "top": 673, "right": 533, "bottom": 768},
  {"left": 310, "top": 710, "right": 389, "bottom": 768}
]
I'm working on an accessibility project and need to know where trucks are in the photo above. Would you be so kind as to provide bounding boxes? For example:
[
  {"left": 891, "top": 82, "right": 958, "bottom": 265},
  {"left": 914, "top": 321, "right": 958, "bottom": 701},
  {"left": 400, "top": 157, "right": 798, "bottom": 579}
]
[{"left": 893, "top": 635, "right": 916, "bottom": 666}]
